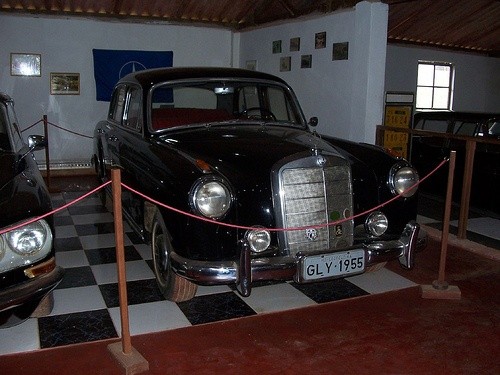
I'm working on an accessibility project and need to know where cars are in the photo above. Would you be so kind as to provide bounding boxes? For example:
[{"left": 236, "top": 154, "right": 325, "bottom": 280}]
[
  {"left": 411, "top": 109, "right": 499, "bottom": 219},
  {"left": 0, "top": 94, "right": 65, "bottom": 329},
  {"left": 91, "top": 64, "right": 421, "bottom": 303}
]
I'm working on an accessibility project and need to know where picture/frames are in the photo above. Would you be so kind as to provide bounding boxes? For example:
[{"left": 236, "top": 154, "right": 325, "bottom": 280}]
[
  {"left": 49, "top": 73, "right": 81, "bottom": 95},
  {"left": 9, "top": 53, "right": 42, "bottom": 77}
]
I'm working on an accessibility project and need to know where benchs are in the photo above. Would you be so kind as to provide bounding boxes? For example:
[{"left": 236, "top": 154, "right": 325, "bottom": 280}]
[{"left": 150, "top": 107, "right": 230, "bottom": 130}]
